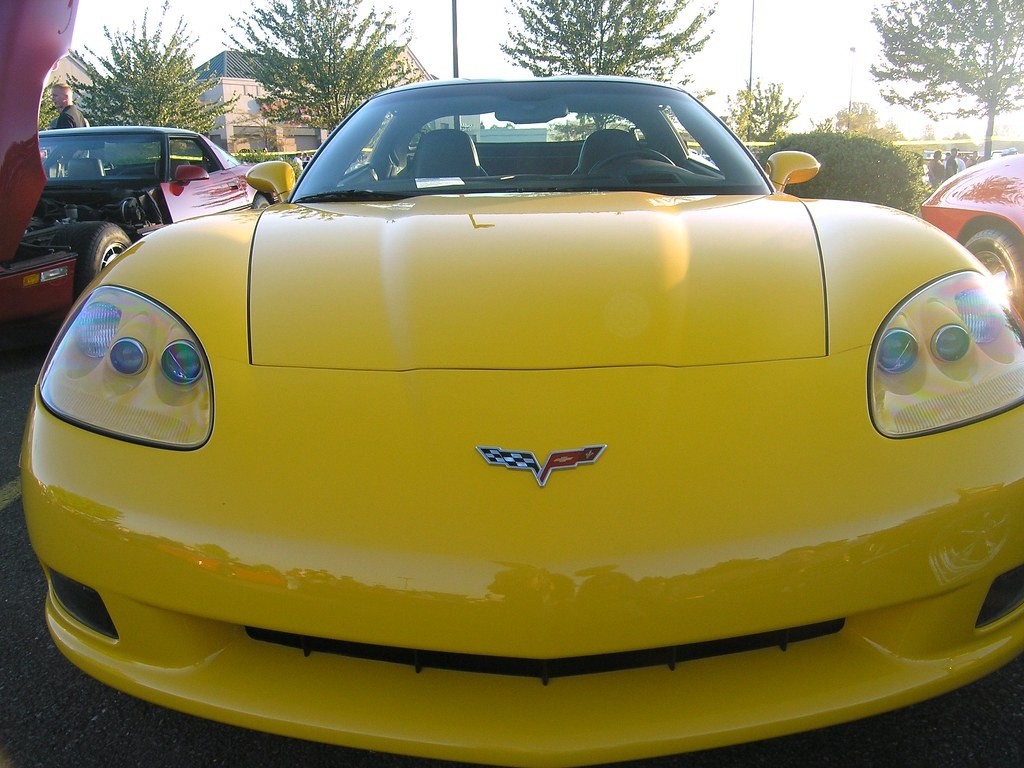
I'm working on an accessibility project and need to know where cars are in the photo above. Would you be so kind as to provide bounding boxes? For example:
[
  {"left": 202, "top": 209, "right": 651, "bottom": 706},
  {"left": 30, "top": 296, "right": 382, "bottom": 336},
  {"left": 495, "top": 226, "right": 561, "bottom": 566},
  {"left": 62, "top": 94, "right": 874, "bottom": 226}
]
[
  {"left": 21, "top": 75, "right": 1023, "bottom": 768},
  {"left": 918, "top": 152, "right": 1024, "bottom": 347},
  {"left": 2, "top": 0, "right": 273, "bottom": 337}
]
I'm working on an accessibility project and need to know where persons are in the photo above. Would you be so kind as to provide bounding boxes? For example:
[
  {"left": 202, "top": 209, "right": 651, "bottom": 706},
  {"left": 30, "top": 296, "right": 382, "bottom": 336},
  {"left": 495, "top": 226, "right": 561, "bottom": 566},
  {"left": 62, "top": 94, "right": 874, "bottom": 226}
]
[
  {"left": 970, "top": 151, "right": 978, "bottom": 166},
  {"left": 945, "top": 147, "right": 959, "bottom": 179},
  {"left": 954, "top": 153, "right": 966, "bottom": 173},
  {"left": 51, "top": 84, "right": 88, "bottom": 129},
  {"left": 943, "top": 152, "right": 951, "bottom": 169},
  {"left": 928, "top": 150, "right": 945, "bottom": 189}
]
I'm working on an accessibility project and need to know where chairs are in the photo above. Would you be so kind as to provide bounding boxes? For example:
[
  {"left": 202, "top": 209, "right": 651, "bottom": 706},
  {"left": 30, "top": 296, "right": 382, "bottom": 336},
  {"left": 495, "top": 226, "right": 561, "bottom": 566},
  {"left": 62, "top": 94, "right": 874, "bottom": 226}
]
[
  {"left": 399, "top": 129, "right": 489, "bottom": 180},
  {"left": 570, "top": 129, "right": 641, "bottom": 176},
  {"left": 68, "top": 157, "right": 105, "bottom": 177},
  {"left": 154, "top": 159, "right": 191, "bottom": 179}
]
